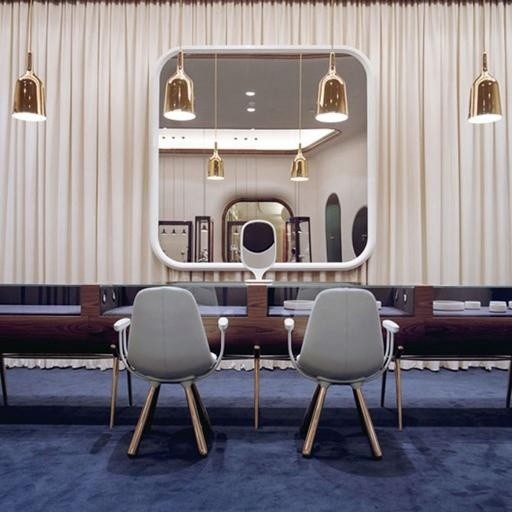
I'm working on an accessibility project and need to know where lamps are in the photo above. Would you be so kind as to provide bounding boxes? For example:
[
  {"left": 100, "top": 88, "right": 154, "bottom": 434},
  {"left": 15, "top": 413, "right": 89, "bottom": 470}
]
[
  {"left": 206, "top": 56, "right": 224, "bottom": 179},
  {"left": 467, "top": 1, "right": 502, "bottom": 126},
  {"left": 312, "top": 1, "right": 348, "bottom": 124},
  {"left": 288, "top": 55, "right": 309, "bottom": 182},
  {"left": 163, "top": 1, "right": 197, "bottom": 121},
  {"left": 10, "top": 1, "right": 49, "bottom": 123}
]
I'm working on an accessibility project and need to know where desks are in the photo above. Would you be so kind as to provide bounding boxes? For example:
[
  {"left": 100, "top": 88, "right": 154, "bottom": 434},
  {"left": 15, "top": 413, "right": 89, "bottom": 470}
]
[{"left": 1, "top": 283, "right": 511, "bottom": 429}]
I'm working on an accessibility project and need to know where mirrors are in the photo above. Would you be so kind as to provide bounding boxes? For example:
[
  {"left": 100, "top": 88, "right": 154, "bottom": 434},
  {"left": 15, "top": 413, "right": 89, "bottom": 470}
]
[{"left": 149, "top": 44, "right": 380, "bottom": 272}]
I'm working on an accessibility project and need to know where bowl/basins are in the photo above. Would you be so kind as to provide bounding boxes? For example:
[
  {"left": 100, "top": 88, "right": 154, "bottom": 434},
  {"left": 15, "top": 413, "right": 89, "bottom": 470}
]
[
  {"left": 433, "top": 299, "right": 511, "bottom": 313},
  {"left": 285, "top": 299, "right": 314, "bottom": 310}
]
